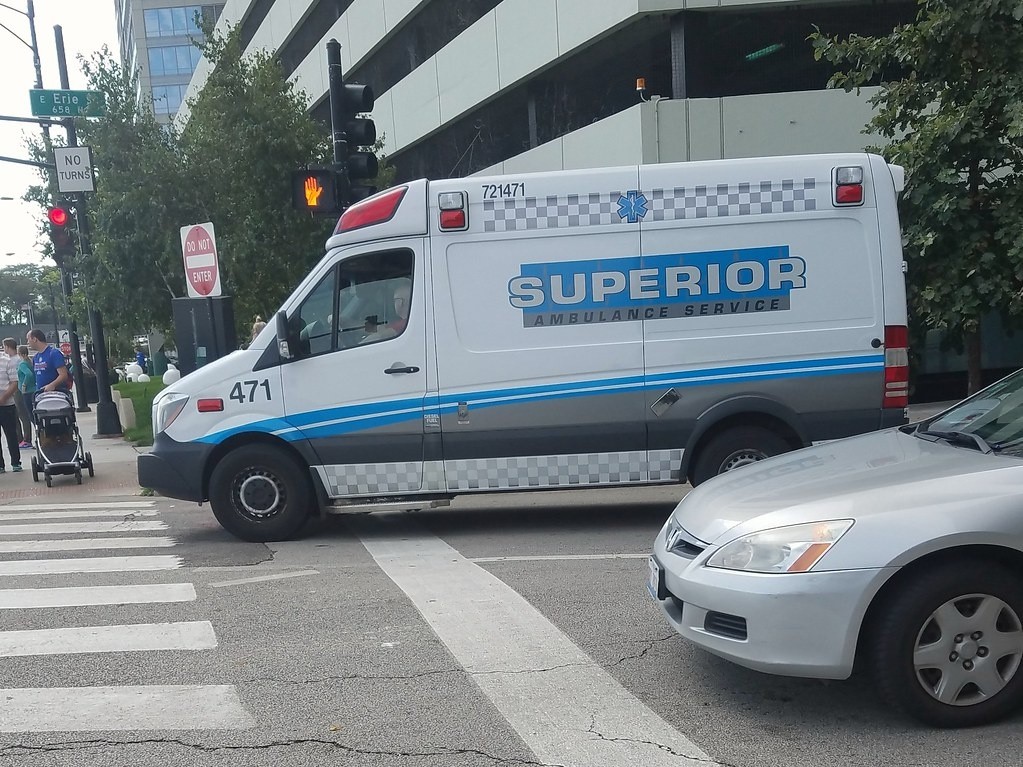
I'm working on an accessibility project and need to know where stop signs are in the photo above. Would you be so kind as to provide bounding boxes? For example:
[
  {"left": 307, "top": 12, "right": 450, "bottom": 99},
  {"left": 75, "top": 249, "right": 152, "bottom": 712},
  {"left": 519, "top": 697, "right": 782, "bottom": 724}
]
[
  {"left": 177, "top": 222, "right": 224, "bottom": 299},
  {"left": 61, "top": 343, "right": 72, "bottom": 355}
]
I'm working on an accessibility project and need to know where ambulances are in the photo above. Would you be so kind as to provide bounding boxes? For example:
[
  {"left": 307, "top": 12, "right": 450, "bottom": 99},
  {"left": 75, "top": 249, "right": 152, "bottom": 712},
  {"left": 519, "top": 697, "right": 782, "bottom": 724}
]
[{"left": 136, "top": 152, "right": 913, "bottom": 544}]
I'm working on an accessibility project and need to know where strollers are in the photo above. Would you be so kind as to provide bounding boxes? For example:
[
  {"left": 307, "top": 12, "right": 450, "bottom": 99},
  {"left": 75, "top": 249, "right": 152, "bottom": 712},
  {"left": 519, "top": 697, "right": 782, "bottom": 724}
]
[{"left": 31, "top": 388, "right": 94, "bottom": 488}]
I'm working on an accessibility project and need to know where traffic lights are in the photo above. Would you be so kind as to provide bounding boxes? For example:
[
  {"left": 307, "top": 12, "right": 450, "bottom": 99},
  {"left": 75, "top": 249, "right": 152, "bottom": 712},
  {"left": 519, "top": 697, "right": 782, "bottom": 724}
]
[
  {"left": 339, "top": 84, "right": 379, "bottom": 208},
  {"left": 291, "top": 169, "right": 339, "bottom": 214},
  {"left": 47, "top": 206, "right": 78, "bottom": 270}
]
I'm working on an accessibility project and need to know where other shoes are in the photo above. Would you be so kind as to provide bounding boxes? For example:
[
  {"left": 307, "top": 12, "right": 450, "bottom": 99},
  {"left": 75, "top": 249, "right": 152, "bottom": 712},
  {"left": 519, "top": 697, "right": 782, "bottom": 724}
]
[
  {"left": 12, "top": 466, "right": 23, "bottom": 472},
  {"left": 0, "top": 468, "right": 5, "bottom": 472}
]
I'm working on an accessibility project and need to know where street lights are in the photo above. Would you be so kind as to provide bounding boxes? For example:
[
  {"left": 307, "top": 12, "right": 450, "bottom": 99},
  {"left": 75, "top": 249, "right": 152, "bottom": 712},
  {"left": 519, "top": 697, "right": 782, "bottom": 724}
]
[
  {"left": 1, "top": 197, "right": 49, "bottom": 210},
  {"left": 27, "top": 299, "right": 39, "bottom": 329},
  {"left": 5, "top": 251, "right": 61, "bottom": 349}
]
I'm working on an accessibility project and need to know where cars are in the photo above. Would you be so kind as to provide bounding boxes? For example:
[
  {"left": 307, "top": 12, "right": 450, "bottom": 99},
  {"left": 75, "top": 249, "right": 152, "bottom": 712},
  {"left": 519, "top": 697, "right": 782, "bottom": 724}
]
[
  {"left": 137, "top": 338, "right": 148, "bottom": 344},
  {"left": 115, "top": 369, "right": 124, "bottom": 382},
  {"left": 646, "top": 369, "right": 1022, "bottom": 726}
]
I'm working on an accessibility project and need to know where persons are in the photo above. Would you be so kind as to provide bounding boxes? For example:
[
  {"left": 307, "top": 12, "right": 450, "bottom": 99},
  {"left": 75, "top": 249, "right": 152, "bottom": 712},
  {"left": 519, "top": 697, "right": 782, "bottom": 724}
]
[
  {"left": 252, "top": 317, "right": 267, "bottom": 343},
  {"left": 0, "top": 328, "right": 68, "bottom": 472},
  {"left": 328, "top": 280, "right": 411, "bottom": 346},
  {"left": 134, "top": 348, "right": 146, "bottom": 371}
]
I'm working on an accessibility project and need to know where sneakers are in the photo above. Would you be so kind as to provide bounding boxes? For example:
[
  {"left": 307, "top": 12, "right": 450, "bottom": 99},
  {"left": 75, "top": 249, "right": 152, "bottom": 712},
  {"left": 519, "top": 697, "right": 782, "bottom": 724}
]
[{"left": 19, "top": 442, "right": 33, "bottom": 449}]
